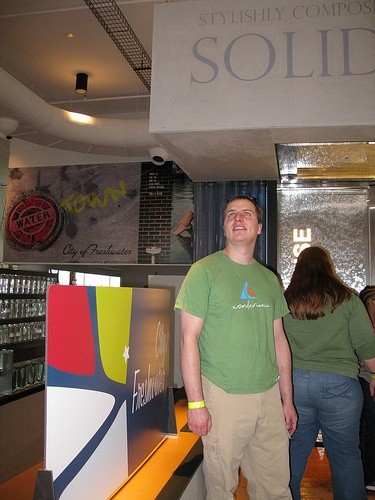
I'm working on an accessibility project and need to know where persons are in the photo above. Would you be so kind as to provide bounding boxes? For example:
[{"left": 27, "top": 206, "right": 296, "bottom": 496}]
[
  {"left": 353, "top": 285, "right": 375, "bottom": 495},
  {"left": 173, "top": 195, "right": 298, "bottom": 500},
  {"left": 282, "top": 246, "right": 375, "bottom": 500}
]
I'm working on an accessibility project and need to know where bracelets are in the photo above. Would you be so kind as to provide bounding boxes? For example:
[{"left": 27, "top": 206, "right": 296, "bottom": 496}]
[
  {"left": 370, "top": 371, "right": 375, "bottom": 374},
  {"left": 367, "top": 375, "right": 375, "bottom": 379},
  {"left": 187, "top": 400, "right": 205, "bottom": 409}
]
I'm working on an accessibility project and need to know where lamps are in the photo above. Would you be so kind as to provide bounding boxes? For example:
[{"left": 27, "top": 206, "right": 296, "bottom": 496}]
[{"left": 75, "top": 73, "right": 88, "bottom": 94}]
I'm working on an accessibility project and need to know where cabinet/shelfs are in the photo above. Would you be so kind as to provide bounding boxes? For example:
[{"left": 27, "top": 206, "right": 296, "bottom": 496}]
[{"left": 0, "top": 268, "right": 58, "bottom": 405}]
